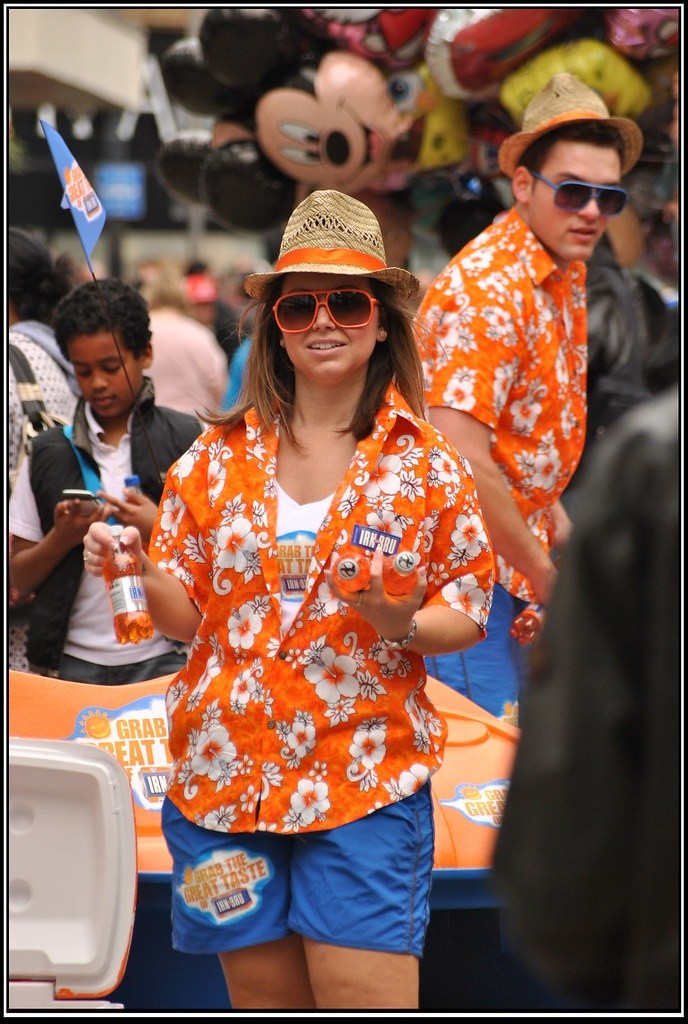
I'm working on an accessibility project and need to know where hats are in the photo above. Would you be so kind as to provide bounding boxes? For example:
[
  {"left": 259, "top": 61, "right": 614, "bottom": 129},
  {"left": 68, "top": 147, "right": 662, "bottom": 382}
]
[
  {"left": 497, "top": 72, "right": 643, "bottom": 178},
  {"left": 242, "top": 189, "right": 420, "bottom": 301}
]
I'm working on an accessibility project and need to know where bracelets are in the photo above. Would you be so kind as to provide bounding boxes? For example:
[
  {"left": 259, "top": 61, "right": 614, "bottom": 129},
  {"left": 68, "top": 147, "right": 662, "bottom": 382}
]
[{"left": 378, "top": 618, "right": 416, "bottom": 650}]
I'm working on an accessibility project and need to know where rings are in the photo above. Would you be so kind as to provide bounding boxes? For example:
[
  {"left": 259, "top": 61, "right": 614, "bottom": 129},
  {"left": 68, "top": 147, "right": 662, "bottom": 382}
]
[
  {"left": 84, "top": 552, "right": 91, "bottom": 560},
  {"left": 356, "top": 592, "right": 361, "bottom": 606}
]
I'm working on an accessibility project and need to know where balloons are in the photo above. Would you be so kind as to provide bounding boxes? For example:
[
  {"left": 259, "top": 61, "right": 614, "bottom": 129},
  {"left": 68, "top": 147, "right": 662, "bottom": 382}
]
[{"left": 158, "top": 7, "right": 682, "bottom": 209}]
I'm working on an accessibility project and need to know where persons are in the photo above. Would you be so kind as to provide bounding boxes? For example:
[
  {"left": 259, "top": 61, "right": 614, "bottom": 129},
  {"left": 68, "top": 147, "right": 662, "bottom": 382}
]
[
  {"left": 8, "top": 278, "right": 204, "bottom": 682},
  {"left": 414, "top": 74, "right": 640, "bottom": 719},
  {"left": 490, "top": 383, "right": 681, "bottom": 1011},
  {"left": 6, "top": 201, "right": 682, "bottom": 679},
  {"left": 83, "top": 190, "right": 495, "bottom": 1010}
]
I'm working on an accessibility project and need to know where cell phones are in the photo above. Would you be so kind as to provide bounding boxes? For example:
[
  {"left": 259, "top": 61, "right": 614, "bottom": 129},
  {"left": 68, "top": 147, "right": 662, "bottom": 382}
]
[{"left": 61, "top": 489, "right": 102, "bottom": 506}]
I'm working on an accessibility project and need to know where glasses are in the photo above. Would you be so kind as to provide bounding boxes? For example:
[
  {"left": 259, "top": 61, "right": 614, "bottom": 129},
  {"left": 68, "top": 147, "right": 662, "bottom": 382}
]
[
  {"left": 527, "top": 168, "right": 628, "bottom": 216},
  {"left": 272, "top": 289, "right": 381, "bottom": 334}
]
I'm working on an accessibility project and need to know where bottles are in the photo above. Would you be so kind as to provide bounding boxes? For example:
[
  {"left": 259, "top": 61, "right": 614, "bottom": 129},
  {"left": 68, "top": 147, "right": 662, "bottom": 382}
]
[
  {"left": 383, "top": 552, "right": 419, "bottom": 597},
  {"left": 101, "top": 517, "right": 154, "bottom": 645},
  {"left": 508, "top": 593, "right": 548, "bottom": 647},
  {"left": 333, "top": 551, "right": 371, "bottom": 595},
  {"left": 121, "top": 475, "right": 145, "bottom": 506}
]
